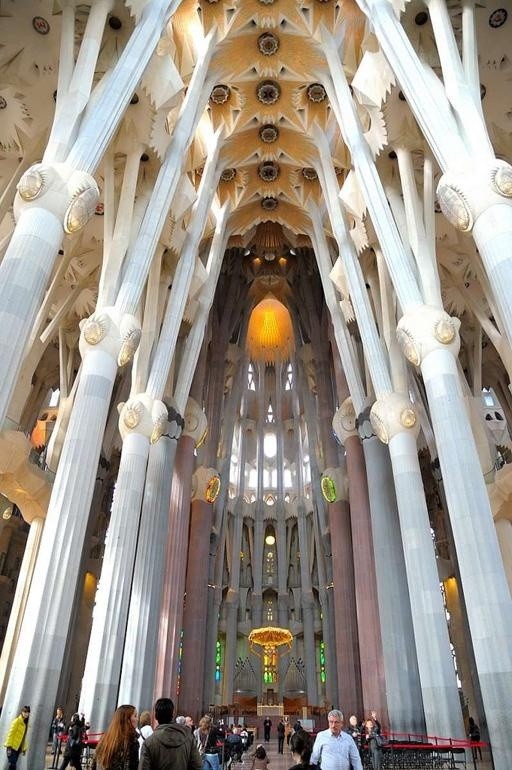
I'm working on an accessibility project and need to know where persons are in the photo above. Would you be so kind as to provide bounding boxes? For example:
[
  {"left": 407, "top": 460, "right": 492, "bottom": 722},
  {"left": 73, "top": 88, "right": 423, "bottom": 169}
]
[
  {"left": 51, "top": 703, "right": 305, "bottom": 770},
  {"left": 343, "top": 715, "right": 366, "bottom": 757},
  {"left": 285, "top": 730, "right": 320, "bottom": 770},
  {"left": 3, "top": 704, "right": 31, "bottom": 770},
  {"left": 364, "top": 710, "right": 387, "bottom": 769},
  {"left": 308, "top": 709, "right": 364, "bottom": 770},
  {"left": 138, "top": 696, "right": 205, "bottom": 770},
  {"left": 466, "top": 716, "right": 483, "bottom": 761}
]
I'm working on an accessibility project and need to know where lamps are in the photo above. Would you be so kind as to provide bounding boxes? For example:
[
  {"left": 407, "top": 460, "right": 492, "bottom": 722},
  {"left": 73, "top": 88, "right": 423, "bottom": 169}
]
[
  {"left": 245, "top": 274, "right": 296, "bottom": 368},
  {"left": 247, "top": 625, "right": 293, "bottom": 685}
]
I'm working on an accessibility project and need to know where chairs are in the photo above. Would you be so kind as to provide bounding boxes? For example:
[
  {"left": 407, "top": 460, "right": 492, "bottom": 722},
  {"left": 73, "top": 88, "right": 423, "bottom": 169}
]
[
  {"left": 201, "top": 730, "right": 254, "bottom": 770},
  {"left": 307, "top": 732, "right": 468, "bottom": 770}
]
